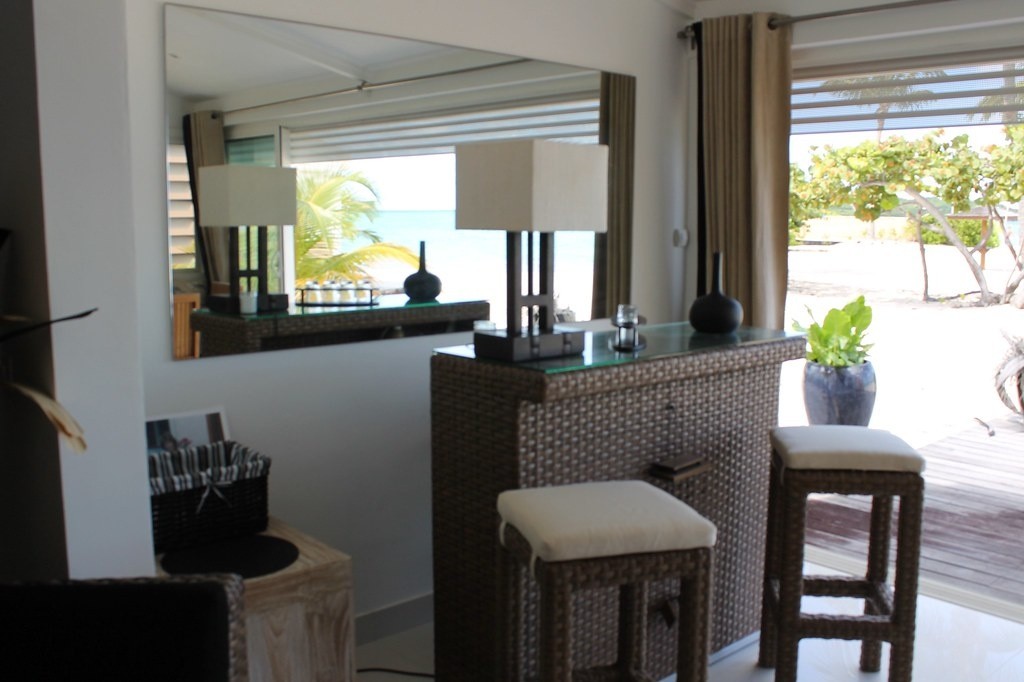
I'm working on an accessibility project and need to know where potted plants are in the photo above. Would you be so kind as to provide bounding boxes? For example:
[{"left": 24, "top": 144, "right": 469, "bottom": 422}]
[{"left": 791, "top": 295, "right": 878, "bottom": 426}]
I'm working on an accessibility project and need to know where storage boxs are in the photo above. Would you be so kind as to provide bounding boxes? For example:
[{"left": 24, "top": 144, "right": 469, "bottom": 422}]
[
  {"left": 156, "top": 522, "right": 359, "bottom": 682},
  {"left": 148, "top": 440, "right": 272, "bottom": 551}
]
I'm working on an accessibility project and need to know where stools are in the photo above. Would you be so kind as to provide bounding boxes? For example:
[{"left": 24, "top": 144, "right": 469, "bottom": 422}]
[
  {"left": 758, "top": 426, "right": 927, "bottom": 682},
  {"left": 498, "top": 480, "right": 718, "bottom": 682}
]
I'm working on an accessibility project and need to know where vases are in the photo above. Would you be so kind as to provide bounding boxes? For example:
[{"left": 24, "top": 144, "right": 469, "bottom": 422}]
[
  {"left": 688, "top": 250, "right": 744, "bottom": 332},
  {"left": 404, "top": 241, "right": 442, "bottom": 298}
]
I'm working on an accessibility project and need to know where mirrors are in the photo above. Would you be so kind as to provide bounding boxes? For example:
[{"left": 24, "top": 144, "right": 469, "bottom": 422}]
[{"left": 163, "top": 4, "right": 638, "bottom": 361}]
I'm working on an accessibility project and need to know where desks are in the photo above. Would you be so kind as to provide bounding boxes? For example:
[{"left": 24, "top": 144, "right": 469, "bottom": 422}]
[
  {"left": 190, "top": 301, "right": 489, "bottom": 358},
  {"left": 430, "top": 321, "right": 807, "bottom": 681}
]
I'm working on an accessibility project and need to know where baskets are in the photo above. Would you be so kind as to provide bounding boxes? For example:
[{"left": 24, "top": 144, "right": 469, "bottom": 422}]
[{"left": 148, "top": 440, "right": 272, "bottom": 551}]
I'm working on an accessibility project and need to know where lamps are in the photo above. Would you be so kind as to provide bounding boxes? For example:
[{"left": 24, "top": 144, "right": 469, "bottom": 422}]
[
  {"left": 456, "top": 138, "right": 609, "bottom": 363},
  {"left": 197, "top": 165, "right": 297, "bottom": 315}
]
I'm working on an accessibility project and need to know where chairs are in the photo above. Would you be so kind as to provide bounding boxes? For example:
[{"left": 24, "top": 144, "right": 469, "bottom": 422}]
[
  {"left": 173, "top": 293, "right": 201, "bottom": 358},
  {"left": 0, "top": 573, "right": 248, "bottom": 681}
]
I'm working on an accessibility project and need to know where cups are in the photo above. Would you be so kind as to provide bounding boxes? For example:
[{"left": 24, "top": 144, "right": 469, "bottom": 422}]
[
  {"left": 615, "top": 304, "right": 638, "bottom": 348},
  {"left": 306, "top": 280, "right": 322, "bottom": 303},
  {"left": 339, "top": 280, "right": 356, "bottom": 303},
  {"left": 324, "top": 280, "right": 338, "bottom": 303},
  {"left": 239, "top": 291, "right": 257, "bottom": 314},
  {"left": 357, "top": 279, "right": 371, "bottom": 303}
]
